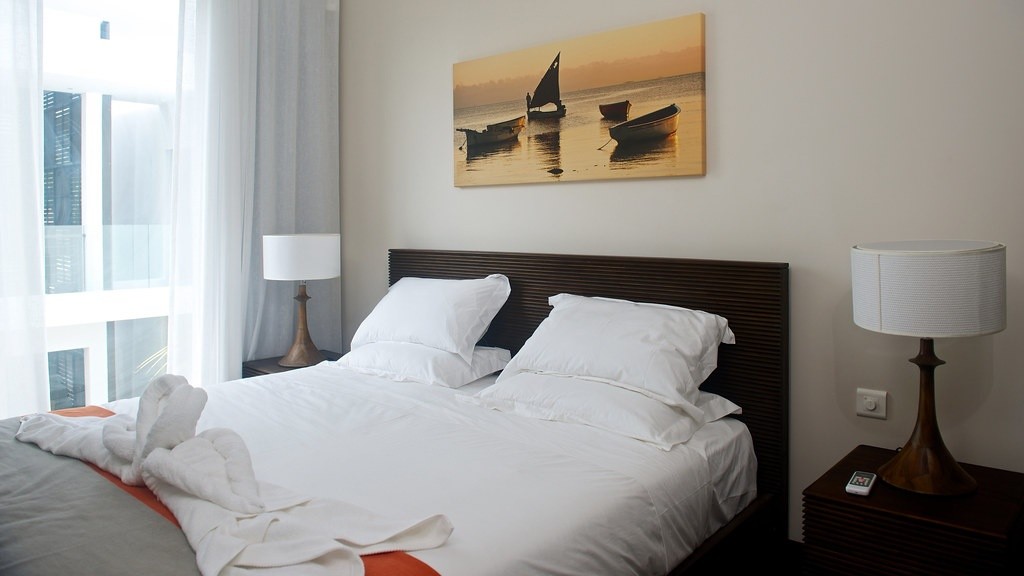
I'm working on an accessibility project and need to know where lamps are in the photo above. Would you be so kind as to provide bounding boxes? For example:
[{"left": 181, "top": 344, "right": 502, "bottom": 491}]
[
  {"left": 850, "top": 241, "right": 1007, "bottom": 495},
  {"left": 262, "top": 234, "right": 340, "bottom": 367}
]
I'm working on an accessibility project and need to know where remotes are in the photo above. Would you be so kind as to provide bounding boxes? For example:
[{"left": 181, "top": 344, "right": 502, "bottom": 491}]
[{"left": 845, "top": 471, "right": 876, "bottom": 495}]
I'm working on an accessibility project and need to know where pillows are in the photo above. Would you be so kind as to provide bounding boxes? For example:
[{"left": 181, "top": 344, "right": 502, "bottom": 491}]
[
  {"left": 472, "top": 371, "right": 742, "bottom": 452},
  {"left": 350, "top": 274, "right": 511, "bottom": 367},
  {"left": 494, "top": 293, "right": 736, "bottom": 423},
  {"left": 336, "top": 340, "right": 511, "bottom": 389}
]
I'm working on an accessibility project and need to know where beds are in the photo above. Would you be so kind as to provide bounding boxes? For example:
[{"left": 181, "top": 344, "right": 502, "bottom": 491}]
[{"left": 0, "top": 247, "right": 791, "bottom": 576}]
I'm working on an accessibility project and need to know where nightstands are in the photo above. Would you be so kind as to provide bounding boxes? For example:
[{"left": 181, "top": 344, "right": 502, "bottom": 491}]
[
  {"left": 242, "top": 349, "right": 345, "bottom": 378},
  {"left": 801, "top": 444, "right": 1023, "bottom": 576}
]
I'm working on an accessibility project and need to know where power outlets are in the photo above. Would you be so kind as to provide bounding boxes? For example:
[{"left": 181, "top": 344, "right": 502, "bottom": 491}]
[{"left": 856, "top": 387, "right": 887, "bottom": 420}]
